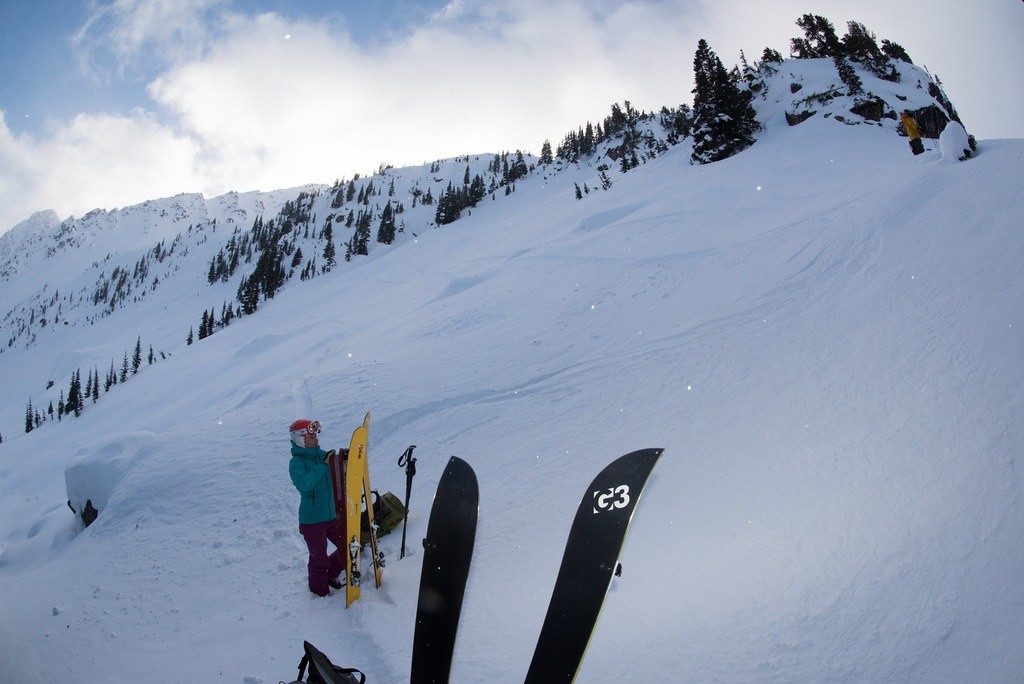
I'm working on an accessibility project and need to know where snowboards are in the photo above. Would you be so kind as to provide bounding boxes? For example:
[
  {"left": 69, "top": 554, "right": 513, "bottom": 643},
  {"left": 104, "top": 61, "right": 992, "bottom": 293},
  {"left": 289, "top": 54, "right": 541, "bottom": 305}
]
[
  {"left": 409, "top": 453, "right": 480, "bottom": 684},
  {"left": 524, "top": 447, "right": 666, "bottom": 684}
]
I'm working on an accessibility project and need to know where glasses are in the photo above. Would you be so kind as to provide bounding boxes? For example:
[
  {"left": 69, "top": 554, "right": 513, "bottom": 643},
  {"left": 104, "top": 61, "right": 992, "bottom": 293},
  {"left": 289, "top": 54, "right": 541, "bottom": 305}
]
[{"left": 307, "top": 420, "right": 320, "bottom": 434}]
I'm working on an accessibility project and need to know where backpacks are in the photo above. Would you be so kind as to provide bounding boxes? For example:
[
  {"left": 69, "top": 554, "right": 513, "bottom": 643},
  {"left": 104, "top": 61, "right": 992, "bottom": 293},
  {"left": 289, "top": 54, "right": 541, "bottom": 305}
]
[{"left": 298, "top": 640, "right": 367, "bottom": 684}]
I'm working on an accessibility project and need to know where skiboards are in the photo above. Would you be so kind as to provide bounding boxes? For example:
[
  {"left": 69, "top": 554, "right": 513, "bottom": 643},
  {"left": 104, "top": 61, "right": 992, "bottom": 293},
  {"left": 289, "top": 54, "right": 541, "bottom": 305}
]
[
  {"left": 342, "top": 427, "right": 363, "bottom": 608},
  {"left": 363, "top": 413, "right": 382, "bottom": 590}
]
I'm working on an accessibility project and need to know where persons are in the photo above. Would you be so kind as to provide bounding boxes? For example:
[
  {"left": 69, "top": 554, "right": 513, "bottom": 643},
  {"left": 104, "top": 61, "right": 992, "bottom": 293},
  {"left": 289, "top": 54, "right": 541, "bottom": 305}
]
[
  {"left": 902, "top": 109, "right": 925, "bottom": 156},
  {"left": 288, "top": 418, "right": 348, "bottom": 597}
]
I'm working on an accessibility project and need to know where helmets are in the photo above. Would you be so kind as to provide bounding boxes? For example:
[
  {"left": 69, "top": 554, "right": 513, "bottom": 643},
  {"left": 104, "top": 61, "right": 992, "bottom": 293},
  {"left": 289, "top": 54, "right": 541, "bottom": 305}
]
[{"left": 289, "top": 419, "right": 321, "bottom": 436}]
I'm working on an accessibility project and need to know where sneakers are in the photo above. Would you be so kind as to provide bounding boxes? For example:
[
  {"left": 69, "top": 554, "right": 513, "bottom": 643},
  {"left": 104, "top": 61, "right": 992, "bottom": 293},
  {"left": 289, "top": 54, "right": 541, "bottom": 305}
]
[{"left": 328, "top": 578, "right": 342, "bottom": 589}]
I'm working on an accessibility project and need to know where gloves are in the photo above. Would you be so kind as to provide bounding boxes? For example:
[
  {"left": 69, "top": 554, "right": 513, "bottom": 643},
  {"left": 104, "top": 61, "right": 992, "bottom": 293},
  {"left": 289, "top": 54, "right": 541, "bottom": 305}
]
[
  {"left": 323, "top": 449, "right": 336, "bottom": 464},
  {"left": 339, "top": 447, "right": 349, "bottom": 460}
]
